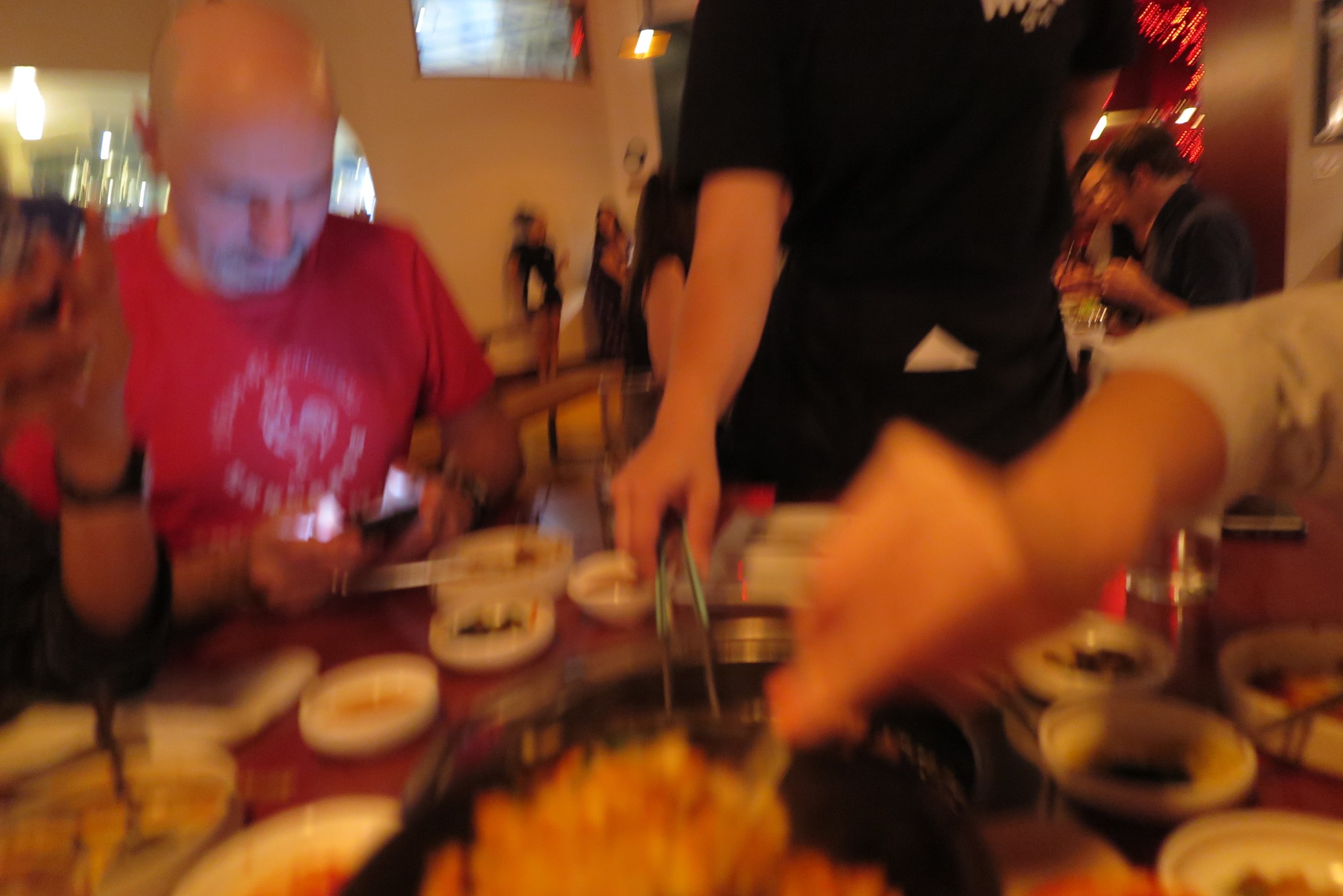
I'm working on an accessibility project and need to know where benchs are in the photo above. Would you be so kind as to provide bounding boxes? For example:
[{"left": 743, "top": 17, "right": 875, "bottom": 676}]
[{"left": 422, "top": 358, "right": 624, "bottom": 481}]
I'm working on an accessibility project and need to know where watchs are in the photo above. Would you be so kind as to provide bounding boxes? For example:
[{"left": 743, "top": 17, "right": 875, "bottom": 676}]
[
  {"left": 448, "top": 468, "right": 490, "bottom": 527},
  {"left": 54, "top": 432, "right": 151, "bottom": 510}
]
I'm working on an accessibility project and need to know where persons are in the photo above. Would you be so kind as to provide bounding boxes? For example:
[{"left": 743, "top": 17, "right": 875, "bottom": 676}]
[
  {"left": 506, "top": 205, "right": 569, "bottom": 383},
  {"left": 770, "top": 281, "right": 1342, "bottom": 745},
  {"left": 618, "top": 164, "right": 693, "bottom": 447},
  {"left": 0, "top": 0, "right": 526, "bottom": 628},
  {"left": 611, "top": 0, "right": 1149, "bottom": 589},
  {"left": 1055, "top": 122, "right": 1260, "bottom": 333},
  {"left": 588, "top": 203, "right": 630, "bottom": 360},
  {"left": 0, "top": 202, "right": 177, "bottom": 732}
]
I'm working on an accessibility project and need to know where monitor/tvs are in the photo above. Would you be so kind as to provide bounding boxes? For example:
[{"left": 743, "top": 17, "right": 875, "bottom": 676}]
[{"left": 409, "top": 0, "right": 588, "bottom": 81}]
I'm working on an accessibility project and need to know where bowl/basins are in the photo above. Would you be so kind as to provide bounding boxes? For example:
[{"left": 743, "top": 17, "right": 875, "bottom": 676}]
[
  {"left": 1219, "top": 622, "right": 1343, "bottom": 779},
  {"left": 717, "top": 502, "right": 843, "bottom": 607},
  {"left": 1157, "top": 809, "right": 1343, "bottom": 896},
  {"left": 1011, "top": 608, "right": 1177, "bottom": 702},
  {"left": 1037, "top": 691, "right": 1260, "bottom": 821},
  {"left": 0, "top": 733, "right": 244, "bottom": 895},
  {"left": 297, "top": 525, "right": 653, "bottom": 755},
  {"left": 169, "top": 789, "right": 402, "bottom": 896}
]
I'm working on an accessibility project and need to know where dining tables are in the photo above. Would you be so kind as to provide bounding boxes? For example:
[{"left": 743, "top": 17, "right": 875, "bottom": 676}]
[{"left": 145, "top": 486, "right": 1342, "bottom": 896}]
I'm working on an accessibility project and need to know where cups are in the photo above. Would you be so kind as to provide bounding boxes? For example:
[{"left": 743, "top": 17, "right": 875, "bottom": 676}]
[{"left": 1130, "top": 504, "right": 1219, "bottom": 605}]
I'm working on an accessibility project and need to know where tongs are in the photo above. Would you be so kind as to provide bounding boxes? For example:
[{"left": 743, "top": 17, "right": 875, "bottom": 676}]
[{"left": 655, "top": 506, "right": 724, "bottom": 730}]
